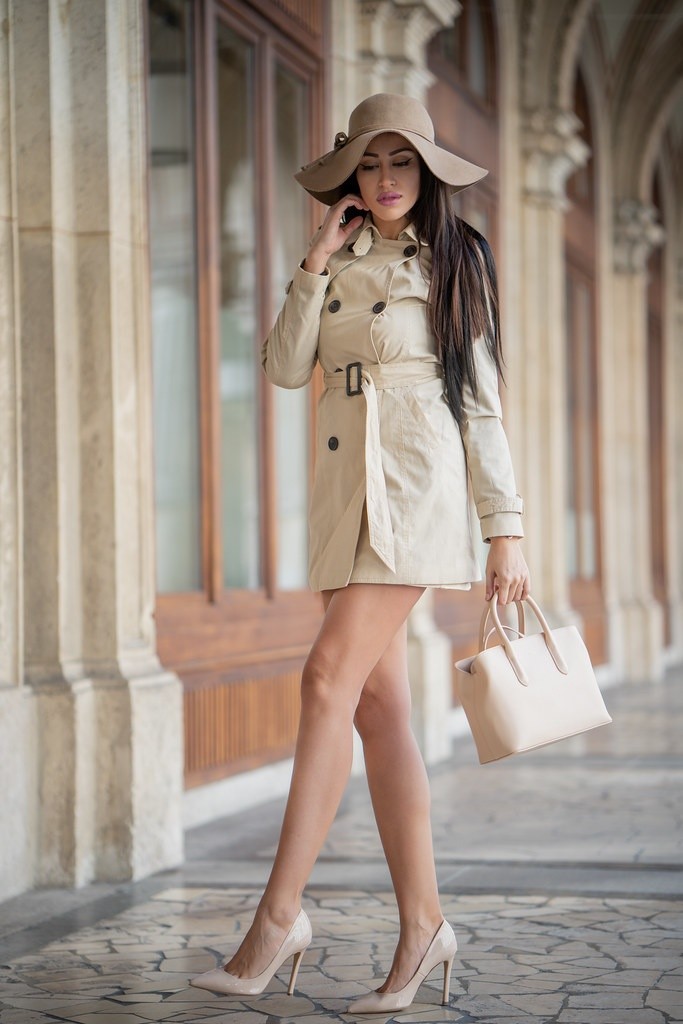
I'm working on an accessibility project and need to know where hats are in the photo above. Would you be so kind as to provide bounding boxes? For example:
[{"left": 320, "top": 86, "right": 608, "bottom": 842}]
[{"left": 292, "top": 92, "right": 490, "bottom": 206}]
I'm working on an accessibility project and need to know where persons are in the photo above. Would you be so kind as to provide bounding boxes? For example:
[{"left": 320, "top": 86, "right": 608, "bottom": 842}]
[{"left": 188, "top": 94, "right": 531, "bottom": 1014}]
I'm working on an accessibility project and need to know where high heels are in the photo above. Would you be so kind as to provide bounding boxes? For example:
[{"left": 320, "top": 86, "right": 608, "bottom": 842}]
[
  {"left": 346, "top": 918, "right": 458, "bottom": 1014},
  {"left": 189, "top": 906, "right": 313, "bottom": 997}
]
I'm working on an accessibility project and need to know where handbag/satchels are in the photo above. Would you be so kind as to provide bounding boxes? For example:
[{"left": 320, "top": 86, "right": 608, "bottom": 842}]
[{"left": 453, "top": 589, "right": 615, "bottom": 766}]
